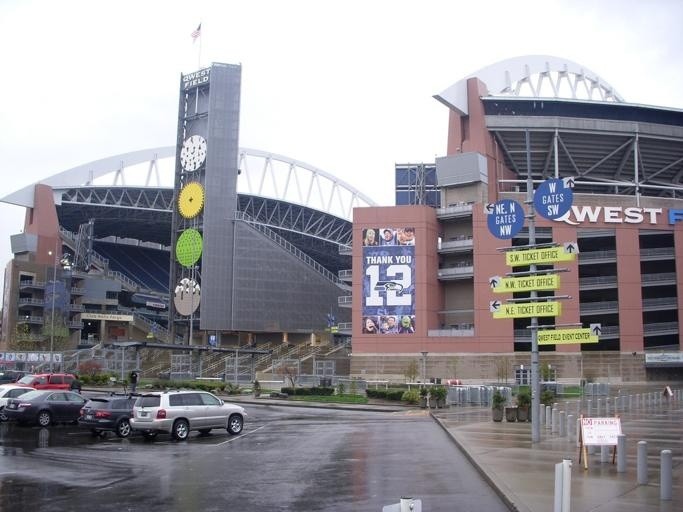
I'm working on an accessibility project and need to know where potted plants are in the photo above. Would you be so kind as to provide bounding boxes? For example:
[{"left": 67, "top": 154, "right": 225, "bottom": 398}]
[
  {"left": 416, "top": 385, "right": 447, "bottom": 409},
  {"left": 489, "top": 386, "right": 556, "bottom": 423}
]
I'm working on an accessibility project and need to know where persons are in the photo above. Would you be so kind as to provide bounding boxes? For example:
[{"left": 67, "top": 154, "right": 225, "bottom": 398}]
[
  {"left": 382, "top": 317, "right": 397, "bottom": 335},
  {"left": 71, "top": 375, "right": 81, "bottom": 394},
  {"left": 381, "top": 229, "right": 397, "bottom": 245},
  {"left": 130, "top": 371, "right": 138, "bottom": 393},
  {"left": 363, "top": 228, "right": 378, "bottom": 246},
  {"left": 363, "top": 319, "right": 379, "bottom": 334}
]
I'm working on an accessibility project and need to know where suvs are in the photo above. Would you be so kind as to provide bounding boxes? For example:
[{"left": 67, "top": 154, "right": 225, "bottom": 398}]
[
  {"left": 0, "top": 370, "right": 33, "bottom": 385},
  {"left": 15, "top": 372, "right": 79, "bottom": 390},
  {"left": 77, "top": 394, "right": 140, "bottom": 440},
  {"left": 130, "top": 389, "right": 247, "bottom": 443}
]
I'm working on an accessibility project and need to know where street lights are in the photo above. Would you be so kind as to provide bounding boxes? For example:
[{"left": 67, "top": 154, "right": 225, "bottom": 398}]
[{"left": 48, "top": 236, "right": 74, "bottom": 372}]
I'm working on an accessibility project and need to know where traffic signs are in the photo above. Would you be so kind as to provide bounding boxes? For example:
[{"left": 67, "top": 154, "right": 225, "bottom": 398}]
[
  {"left": 485, "top": 273, "right": 559, "bottom": 294},
  {"left": 533, "top": 175, "right": 576, "bottom": 220},
  {"left": 535, "top": 322, "right": 602, "bottom": 345},
  {"left": 489, "top": 299, "right": 562, "bottom": 320},
  {"left": 483, "top": 199, "right": 525, "bottom": 239},
  {"left": 505, "top": 241, "right": 579, "bottom": 267}
]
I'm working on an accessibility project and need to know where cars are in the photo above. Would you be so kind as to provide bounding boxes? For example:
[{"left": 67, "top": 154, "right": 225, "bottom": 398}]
[
  {"left": 5, "top": 389, "right": 87, "bottom": 428},
  {"left": 0, "top": 384, "right": 37, "bottom": 422}
]
